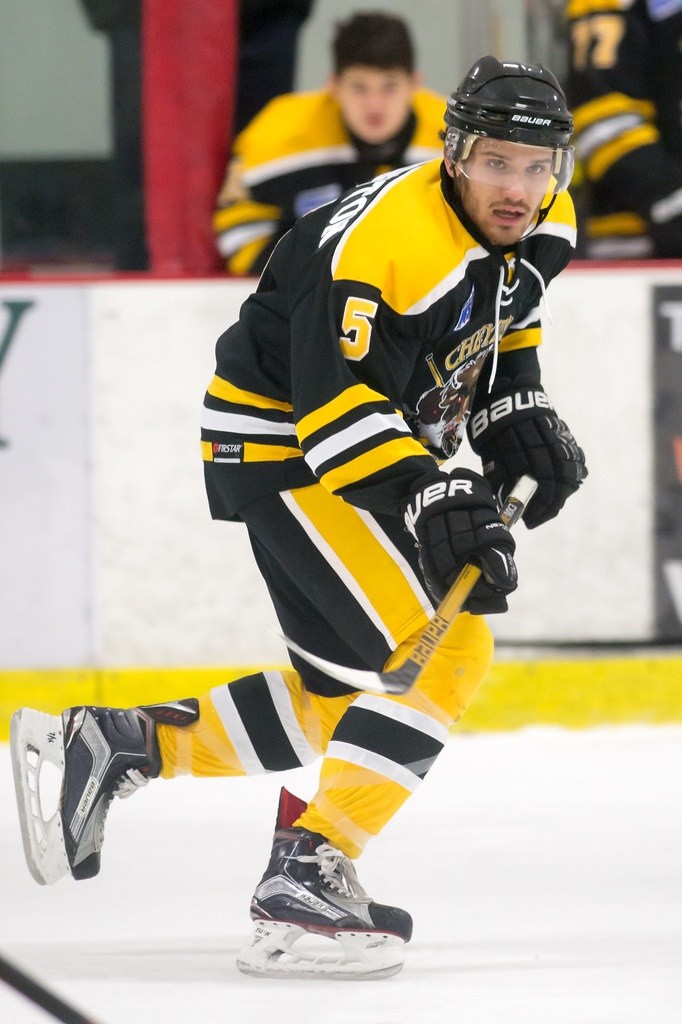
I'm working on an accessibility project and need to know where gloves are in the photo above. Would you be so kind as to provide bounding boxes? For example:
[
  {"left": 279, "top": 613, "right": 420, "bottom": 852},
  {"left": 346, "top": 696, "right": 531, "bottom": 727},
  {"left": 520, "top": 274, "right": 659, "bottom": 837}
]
[
  {"left": 401, "top": 468, "right": 520, "bottom": 616},
  {"left": 471, "top": 377, "right": 587, "bottom": 530}
]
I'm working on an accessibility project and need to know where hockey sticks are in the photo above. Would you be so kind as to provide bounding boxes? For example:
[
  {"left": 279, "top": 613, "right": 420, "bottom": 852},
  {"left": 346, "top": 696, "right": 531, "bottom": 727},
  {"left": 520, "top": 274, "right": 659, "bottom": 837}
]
[
  {"left": 0, "top": 954, "right": 100, "bottom": 1024},
  {"left": 274, "top": 472, "right": 539, "bottom": 697}
]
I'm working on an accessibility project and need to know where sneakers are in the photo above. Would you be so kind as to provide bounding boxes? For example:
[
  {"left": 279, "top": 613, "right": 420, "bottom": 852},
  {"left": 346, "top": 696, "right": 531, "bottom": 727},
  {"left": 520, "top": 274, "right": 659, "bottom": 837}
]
[
  {"left": 237, "top": 786, "right": 415, "bottom": 982},
  {"left": 13, "top": 697, "right": 200, "bottom": 883}
]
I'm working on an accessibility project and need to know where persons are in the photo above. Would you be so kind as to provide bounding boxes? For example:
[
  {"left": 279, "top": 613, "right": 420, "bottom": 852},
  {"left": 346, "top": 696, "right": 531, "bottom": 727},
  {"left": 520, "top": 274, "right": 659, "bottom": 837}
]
[
  {"left": 211, "top": 0, "right": 682, "bottom": 267},
  {"left": 61, "top": 56, "right": 589, "bottom": 943}
]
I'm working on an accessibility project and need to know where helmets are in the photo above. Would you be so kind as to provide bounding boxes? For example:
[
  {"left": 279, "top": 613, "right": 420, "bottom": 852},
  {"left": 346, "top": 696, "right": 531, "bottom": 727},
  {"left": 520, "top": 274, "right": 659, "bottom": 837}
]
[{"left": 443, "top": 52, "right": 574, "bottom": 185}]
